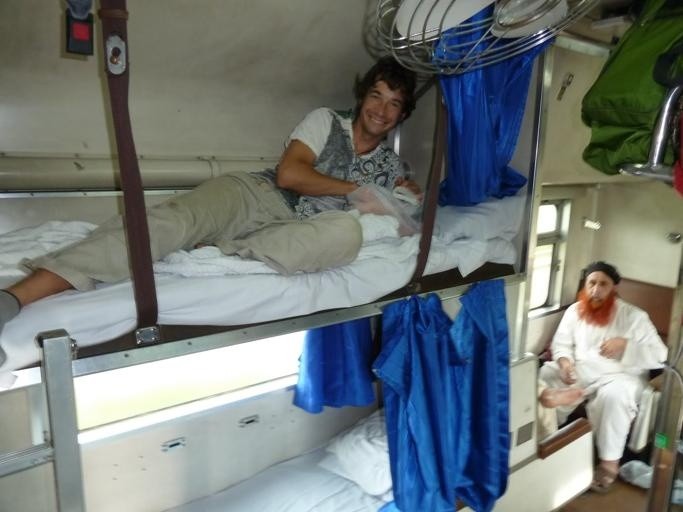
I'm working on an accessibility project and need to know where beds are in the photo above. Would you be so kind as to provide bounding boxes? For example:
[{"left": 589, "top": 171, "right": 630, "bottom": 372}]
[
  {"left": 0, "top": 223, "right": 515, "bottom": 393},
  {"left": 167, "top": 408, "right": 479, "bottom": 512}
]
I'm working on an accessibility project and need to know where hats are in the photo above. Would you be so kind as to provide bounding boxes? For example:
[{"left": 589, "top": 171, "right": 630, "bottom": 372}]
[{"left": 585, "top": 260, "right": 620, "bottom": 288}]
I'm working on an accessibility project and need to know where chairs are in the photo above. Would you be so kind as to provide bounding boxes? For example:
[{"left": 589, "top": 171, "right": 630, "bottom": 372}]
[{"left": 535, "top": 269, "right": 676, "bottom": 462}]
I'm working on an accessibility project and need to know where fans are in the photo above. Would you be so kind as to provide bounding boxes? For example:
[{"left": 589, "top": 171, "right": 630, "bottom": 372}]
[{"left": 375, "top": 0, "right": 599, "bottom": 76}]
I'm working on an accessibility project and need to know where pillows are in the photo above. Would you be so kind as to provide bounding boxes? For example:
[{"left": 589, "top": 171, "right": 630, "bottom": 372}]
[
  {"left": 325, "top": 412, "right": 394, "bottom": 498},
  {"left": 409, "top": 145, "right": 519, "bottom": 244}
]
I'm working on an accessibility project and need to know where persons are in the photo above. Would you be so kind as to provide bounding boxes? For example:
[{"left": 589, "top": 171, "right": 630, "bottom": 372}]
[
  {"left": 537, "top": 379, "right": 583, "bottom": 442},
  {"left": 539, "top": 261, "right": 668, "bottom": 496},
  {"left": 0, "top": 55, "right": 424, "bottom": 369}
]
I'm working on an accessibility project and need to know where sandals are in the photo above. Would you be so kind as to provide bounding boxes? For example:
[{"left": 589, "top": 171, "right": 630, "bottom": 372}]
[{"left": 592, "top": 468, "right": 617, "bottom": 494}]
